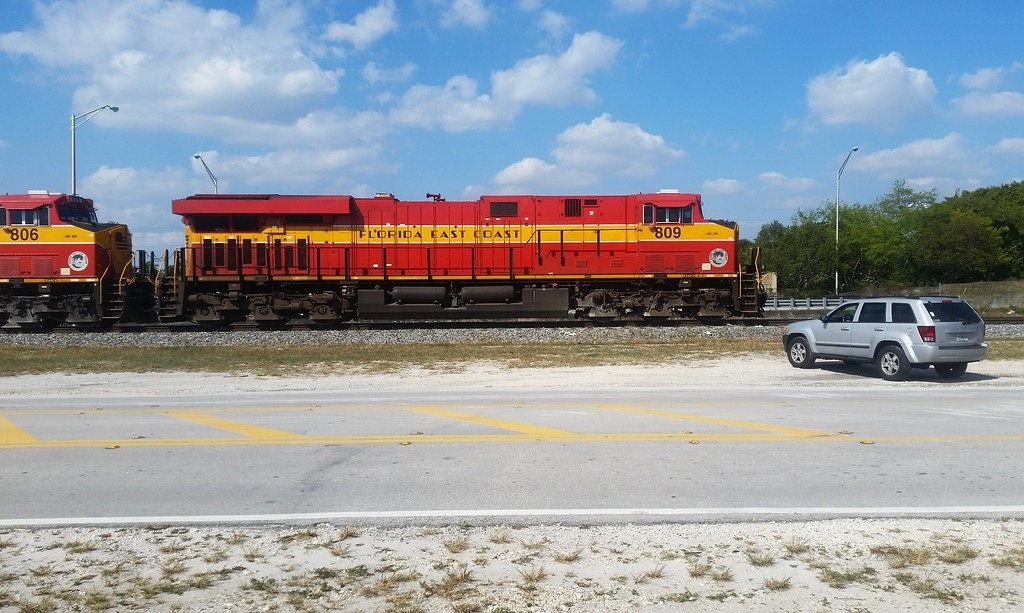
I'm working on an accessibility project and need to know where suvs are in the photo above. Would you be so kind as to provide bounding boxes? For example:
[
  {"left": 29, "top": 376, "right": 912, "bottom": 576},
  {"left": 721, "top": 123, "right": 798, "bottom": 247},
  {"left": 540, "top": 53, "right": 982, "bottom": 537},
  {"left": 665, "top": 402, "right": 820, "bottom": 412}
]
[{"left": 781, "top": 295, "right": 988, "bottom": 382}]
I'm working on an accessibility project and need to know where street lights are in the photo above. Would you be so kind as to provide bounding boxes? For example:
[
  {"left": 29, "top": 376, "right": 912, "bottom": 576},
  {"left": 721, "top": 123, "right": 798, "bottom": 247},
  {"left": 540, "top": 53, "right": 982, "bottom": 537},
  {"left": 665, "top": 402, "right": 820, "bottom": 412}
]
[
  {"left": 71, "top": 104, "right": 120, "bottom": 195},
  {"left": 834, "top": 146, "right": 859, "bottom": 296},
  {"left": 193, "top": 154, "right": 218, "bottom": 195}
]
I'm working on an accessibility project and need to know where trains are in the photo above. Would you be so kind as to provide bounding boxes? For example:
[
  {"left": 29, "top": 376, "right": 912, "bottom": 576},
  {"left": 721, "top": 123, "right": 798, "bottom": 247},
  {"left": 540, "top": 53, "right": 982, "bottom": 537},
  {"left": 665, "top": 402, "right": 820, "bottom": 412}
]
[{"left": 0, "top": 188, "right": 767, "bottom": 332}]
